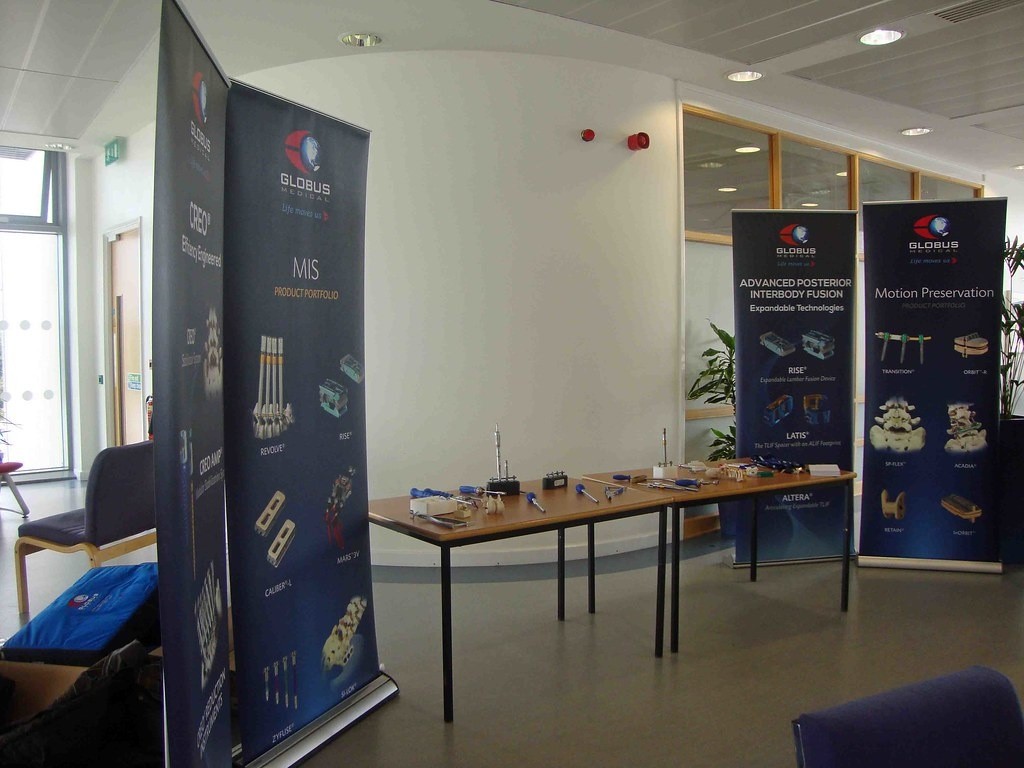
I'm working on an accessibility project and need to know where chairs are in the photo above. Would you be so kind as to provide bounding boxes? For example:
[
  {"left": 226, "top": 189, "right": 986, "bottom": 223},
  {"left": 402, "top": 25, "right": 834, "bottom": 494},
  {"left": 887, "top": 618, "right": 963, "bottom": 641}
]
[
  {"left": 14, "top": 441, "right": 157, "bottom": 613},
  {"left": 792, "top": 665, "right": 1024, "bottom": 768}
]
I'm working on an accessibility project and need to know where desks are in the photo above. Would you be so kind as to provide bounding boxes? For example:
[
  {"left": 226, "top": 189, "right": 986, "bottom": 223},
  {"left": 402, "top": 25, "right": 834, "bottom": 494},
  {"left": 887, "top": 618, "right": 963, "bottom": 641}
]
[
  {"left": 368, "top": 476, "right": 674, "bottom": 723},
  {"left": 582, "top": 459, "right": 857, "bottom": 658}
]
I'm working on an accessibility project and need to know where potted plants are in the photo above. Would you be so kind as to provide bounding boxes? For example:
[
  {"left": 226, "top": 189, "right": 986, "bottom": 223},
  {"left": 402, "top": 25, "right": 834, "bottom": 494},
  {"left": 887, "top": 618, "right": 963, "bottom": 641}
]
[
  {"left": 997, "top": 246, "right": 1024, "bottom": 567},
  {"left": 686, "top": 316, "right": 737, "bottom": 535}
]
